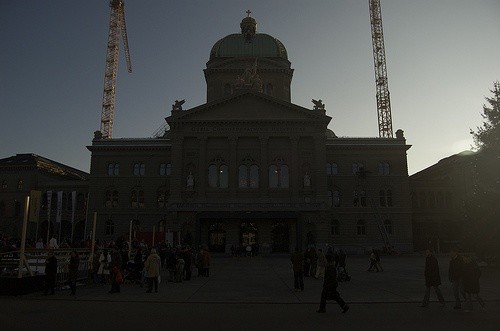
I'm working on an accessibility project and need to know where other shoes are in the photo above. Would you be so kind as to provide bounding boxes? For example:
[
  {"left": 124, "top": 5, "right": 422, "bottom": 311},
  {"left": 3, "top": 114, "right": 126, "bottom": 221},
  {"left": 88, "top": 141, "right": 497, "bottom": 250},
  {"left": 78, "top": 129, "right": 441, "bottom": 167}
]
[
  {"left": 422, "top": 301, "right": 486, "bottom": 311},
  {"left": 342, "top": 305, "right": 349, "bottom": 313},
  {"left": 316, "top": 310, "right": 326, "bottom": 312}
]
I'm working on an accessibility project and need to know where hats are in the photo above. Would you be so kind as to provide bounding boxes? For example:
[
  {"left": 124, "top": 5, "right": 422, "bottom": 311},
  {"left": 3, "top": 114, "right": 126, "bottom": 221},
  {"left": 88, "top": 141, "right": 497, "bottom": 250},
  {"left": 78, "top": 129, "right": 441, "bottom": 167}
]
[{"left": 46, "top": 247, "right": 54, "bottom": 251}]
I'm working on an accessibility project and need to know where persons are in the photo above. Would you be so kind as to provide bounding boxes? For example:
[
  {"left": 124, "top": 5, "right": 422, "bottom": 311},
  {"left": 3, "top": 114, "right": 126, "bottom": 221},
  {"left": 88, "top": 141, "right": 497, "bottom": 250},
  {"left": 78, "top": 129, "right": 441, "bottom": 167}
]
[
  {"left": 41, "top": 251, "right": 57, "bottom": 300},
  {"left": 461, "top": 254, "right": 487, "bottom": 313},
  {"left": 134, "top": 249, "right": 143, "bottom": 279},
  {"left": 291, "top": 248, "right": 305, "bottom": 292},
  {"left": 122, "top": 251, "right": 131, "bottom": 280},
  {"left": 68, "top": 251, "right": 80, "bottom": 297},
  {"left": 0, "top": 235, "right": 58, "bottom": 272},
  {"left": 303, "top": 246, "right": 347, "bottom": 280},
  {"left": 144, "top": 248, "right": 161, "bottom": 294},
  {"left": 447, "top": 248, "right": 468, "bottom": 310},
  {"left": 87, "top": 236, "right": 211, "bottom": 285},
  {"left": 107, "top": 252, "right": 121, "bottom": 294},
  {"left": 418, "top": 248, "right": 448, "bottom": 308},
  {"left": 315, "top": 252, "right": 351, "bottom": 314},
  {"left": 368, "top": 248, "right": 385, "bottom": 272},
  {"left": 231, "top": 240, "right": 261, "bottom": 258}
]
